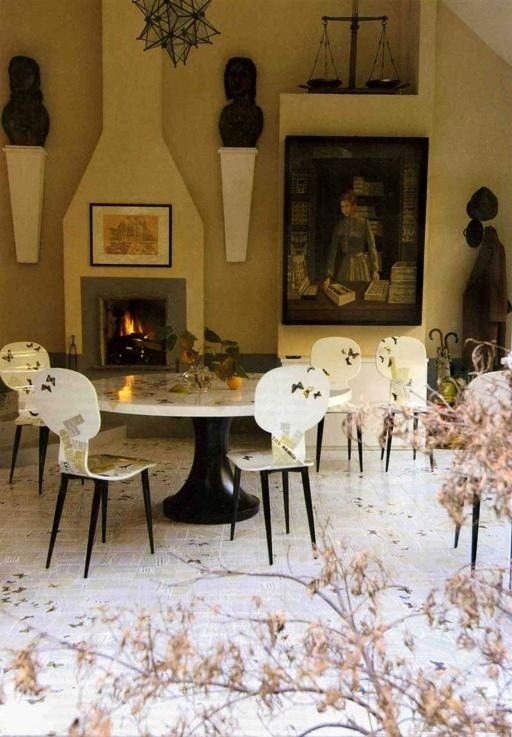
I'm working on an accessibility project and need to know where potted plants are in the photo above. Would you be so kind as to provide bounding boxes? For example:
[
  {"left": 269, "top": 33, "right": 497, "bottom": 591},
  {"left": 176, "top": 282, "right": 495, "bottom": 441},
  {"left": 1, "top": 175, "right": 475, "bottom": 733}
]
[
  {"left": 203, "top": 325, "right": 250, "bottom": 390},
  {"left": 154, "top": 319, "right": 211, "bottom": 392}
]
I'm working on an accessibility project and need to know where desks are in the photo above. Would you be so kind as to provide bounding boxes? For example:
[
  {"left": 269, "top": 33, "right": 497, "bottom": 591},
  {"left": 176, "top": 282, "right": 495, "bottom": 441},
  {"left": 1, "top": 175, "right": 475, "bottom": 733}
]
[{"left": 78, "top": 372, "right": 293, "bottom": 524}]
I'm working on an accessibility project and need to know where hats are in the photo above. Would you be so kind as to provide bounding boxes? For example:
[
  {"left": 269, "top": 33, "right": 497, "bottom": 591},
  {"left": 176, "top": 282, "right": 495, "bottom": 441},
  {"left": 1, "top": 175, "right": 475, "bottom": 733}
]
[
  {"left": 467, "top": 186, "right": 498, "bottom": 220},
  {"left": 466, "top": 220, "right": 483, "bottom": 248}
]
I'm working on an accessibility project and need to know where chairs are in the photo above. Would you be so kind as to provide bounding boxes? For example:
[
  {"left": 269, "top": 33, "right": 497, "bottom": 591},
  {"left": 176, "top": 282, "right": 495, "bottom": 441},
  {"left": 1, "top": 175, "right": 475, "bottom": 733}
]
[
  {"left": 375, "top": 336, "right": 434, "bottom": 472},
  {"left": 226, "top": 365, "right": 330, "bottom": 565},
  {"left": 33, "top": 367, "right": 155, "bottom": 578},
  {"left": 0, "top": 341, "right": 50, "bottom": 495},
  {"left": 455, "top": 370, "right": 512, "bottom": 580},
  {"left": 311, "top": 336, "right": 363, "bottom": 473}
]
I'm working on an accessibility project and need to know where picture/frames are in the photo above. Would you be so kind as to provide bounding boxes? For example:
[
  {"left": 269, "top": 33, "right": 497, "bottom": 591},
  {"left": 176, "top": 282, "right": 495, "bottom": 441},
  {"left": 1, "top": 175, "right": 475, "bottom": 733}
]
[
  {"left": 282, "top": 135, "right": 429, "bottom": 326},
  {"left": 89, "top": 203, "right": 173, "bottom": 267}
]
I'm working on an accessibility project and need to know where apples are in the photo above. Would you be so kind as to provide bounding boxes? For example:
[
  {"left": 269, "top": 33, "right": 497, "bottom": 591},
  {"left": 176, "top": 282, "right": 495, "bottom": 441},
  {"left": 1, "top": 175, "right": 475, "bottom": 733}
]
[
  {"left": 181, "top": 349, "right": 195, "bottom": 364},
  {"left": 226, "top": 374, "right": 241, "bottom": 389}
]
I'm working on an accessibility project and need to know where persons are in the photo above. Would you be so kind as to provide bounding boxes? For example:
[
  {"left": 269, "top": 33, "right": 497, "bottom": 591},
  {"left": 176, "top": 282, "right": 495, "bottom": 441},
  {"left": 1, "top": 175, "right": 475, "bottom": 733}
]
[{"left": 321, "top": 190, "right": 380, "bottom": 292}]
